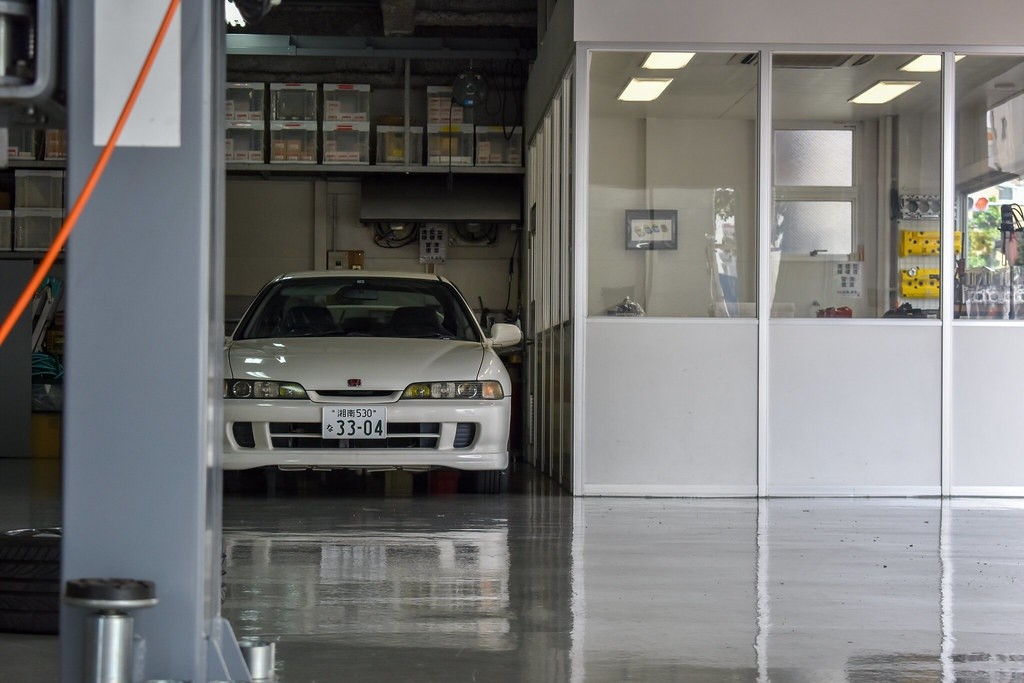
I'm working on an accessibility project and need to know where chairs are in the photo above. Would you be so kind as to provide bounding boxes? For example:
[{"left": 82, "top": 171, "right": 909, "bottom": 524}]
[
  {"left": 386, "top": 306, "right": 440, "bottom": 334},
  {"left": 281, "top": 306, "right": 332, "bottom": 334}
]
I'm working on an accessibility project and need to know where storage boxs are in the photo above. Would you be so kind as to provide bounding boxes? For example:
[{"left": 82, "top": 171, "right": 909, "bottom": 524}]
[
  {"left": 427, "top": 85, "right": 474, "bottom": 124},
  {"left": 225, "top": 123, "right": 265, "bottom": 163},
  {"left": 8, "top": 128, "right": 39, "bottom": 161},
  {"left": 475, "top": 125, "right": 524, "bottom": 165},
  {"left": 269, "top": 82, "right": 318, "bottom": 121},
  {"left": 375, "top": 125, "right": 423, "bottom": 166},
  {"left": 14, "top": 170, "right": 64, "bottom": 207},
  {"left": 323, "top": 84, "right": 371, "bottom": 121},
  {"left": 323, "top": 122, "right": 370, "bottom": 166},
  {"left": 426, "top": 123, "right": 474, "bottom": 167},
  {"left": 44, "top": 128, "right": 67, "bottom": 161},
  {"left": 32, "top": 414, "right": 60, "bottom": 457},
  {"left": 0, "top": 209, "right": 13, "bottom": 251},
  {"left": 269, "top": 121, "right": 318, "bottom": 164},
  {"left": 225, "top": 82, "right": 264, "bottom": 123},
  {"left": 14, "top": 208, "right": 63, "bottom": 252}
]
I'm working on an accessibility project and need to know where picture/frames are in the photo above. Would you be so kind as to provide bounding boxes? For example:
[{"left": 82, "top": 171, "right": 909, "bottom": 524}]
[{"left": 624, "top": 210, "right": 678, "bottom": 249}]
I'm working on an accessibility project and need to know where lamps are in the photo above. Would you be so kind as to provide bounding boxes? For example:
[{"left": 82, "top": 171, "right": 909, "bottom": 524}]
[
  {"left": 895, "top": 55, "right": 967, "bottom": 72},
  {"left": 847, "top": 80, "right": 920, "bottom": 104},
  {"left": 640, "top": 51, "right": 696, "bottom": 70},
  {"left": 615, "top": 76, "right": 673, "bottom": 101}
]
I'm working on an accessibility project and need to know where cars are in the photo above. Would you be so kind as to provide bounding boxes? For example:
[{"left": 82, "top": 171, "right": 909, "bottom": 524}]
[{"left": 218, "top": 269, "right": 526, "bottom": 497}]
